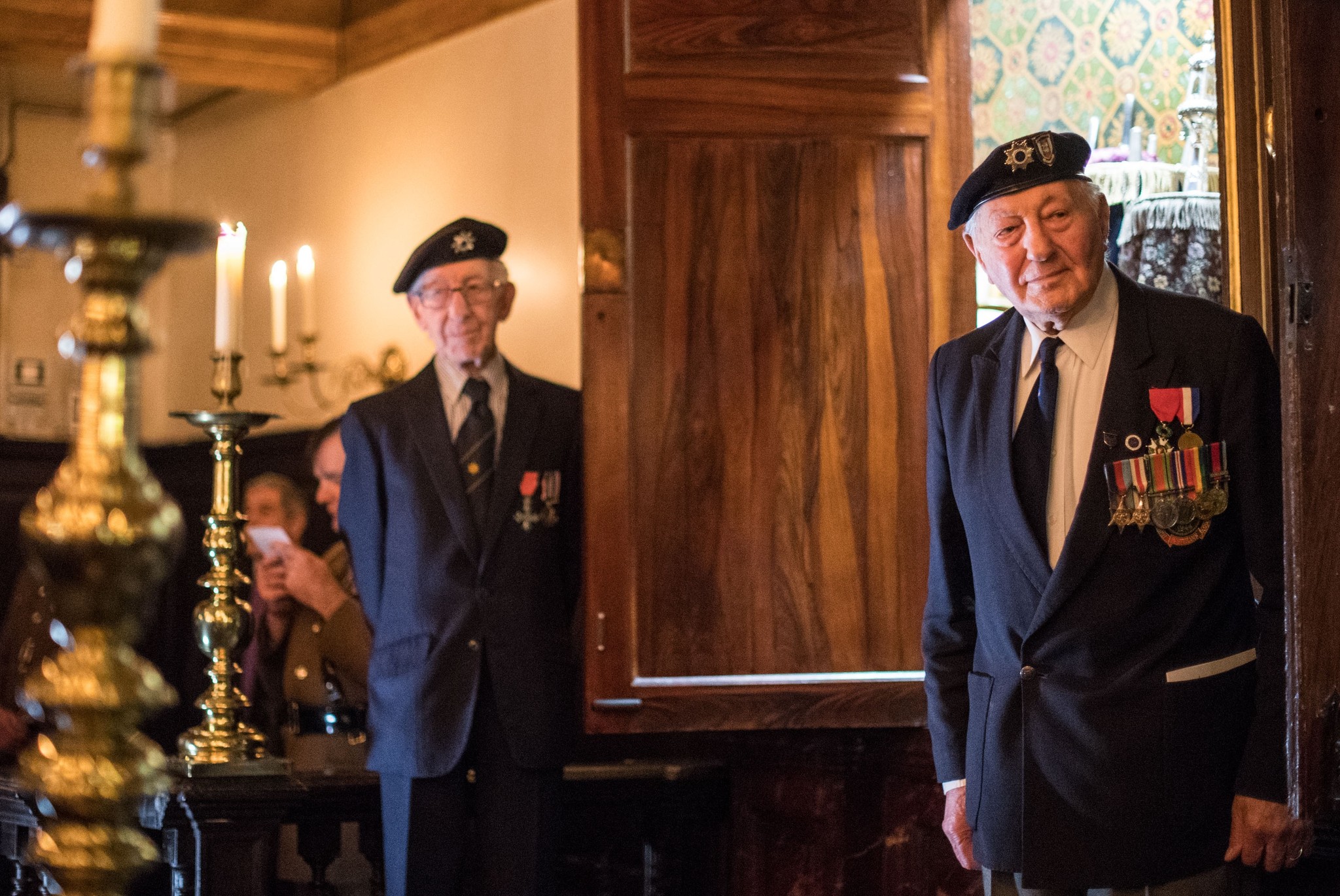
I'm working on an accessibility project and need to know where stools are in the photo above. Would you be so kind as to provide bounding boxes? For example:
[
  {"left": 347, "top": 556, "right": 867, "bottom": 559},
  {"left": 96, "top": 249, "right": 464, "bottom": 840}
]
[{"left": 1116, "top": 191, "right": 1223, "bottom": 303}]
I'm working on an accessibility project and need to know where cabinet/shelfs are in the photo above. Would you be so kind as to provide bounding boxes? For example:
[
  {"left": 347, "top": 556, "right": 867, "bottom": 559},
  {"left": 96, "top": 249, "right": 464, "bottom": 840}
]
[{"left": 567, "top": 756, "right": 724, "bottom": 896}]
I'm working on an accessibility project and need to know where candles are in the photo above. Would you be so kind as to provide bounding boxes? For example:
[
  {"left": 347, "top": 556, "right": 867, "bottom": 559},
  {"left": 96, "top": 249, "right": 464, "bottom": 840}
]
[
  {"left": 269, "top": 260, "right": 289, "bottom": 355},
  {"left": 86, "top": 0, "right": 161, "bottom": 58},
  {"left": 214, "top": 218, "right": 249, "bottom": 353},
  {"left": 296, "top": 245, "right": 316, "bottom": 340}
]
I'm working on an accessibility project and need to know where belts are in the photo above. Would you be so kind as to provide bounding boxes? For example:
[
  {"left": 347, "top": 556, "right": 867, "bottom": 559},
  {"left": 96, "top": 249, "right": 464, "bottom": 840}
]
[{"left": 281, "top": 701, "right": 368, "bottom": 735}]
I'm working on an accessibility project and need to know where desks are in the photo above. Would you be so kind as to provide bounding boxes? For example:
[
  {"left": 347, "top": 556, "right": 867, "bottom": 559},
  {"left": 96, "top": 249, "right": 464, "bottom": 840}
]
[
  {"left": 168, "top": 773, "right": 381, "bottom": 896},
  {"left": 1083, "top": 160, "right": 1220, "bottom": 268}
]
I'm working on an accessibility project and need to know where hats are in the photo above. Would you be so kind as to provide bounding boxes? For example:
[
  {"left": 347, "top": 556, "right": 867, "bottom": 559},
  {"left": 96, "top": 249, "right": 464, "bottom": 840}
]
[
  {"left": 394, "top": 218, "right": 507, "bottom": 293},
  {"left": 947, "top": 130, "right": 1093, "bottom": 230}
]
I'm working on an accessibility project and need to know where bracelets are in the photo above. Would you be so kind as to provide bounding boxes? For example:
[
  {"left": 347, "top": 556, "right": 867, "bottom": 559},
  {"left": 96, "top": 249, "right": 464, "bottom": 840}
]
[{"left": 267, "top": 603, "right": 300, "bottom": 619}]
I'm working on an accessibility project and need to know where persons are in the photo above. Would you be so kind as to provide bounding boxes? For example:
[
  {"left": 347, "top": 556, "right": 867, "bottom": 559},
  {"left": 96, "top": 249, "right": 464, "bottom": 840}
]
[
  {"left": 338, "top": 217, "right": 583, "bottom": 896},
  {"left": 920, "top": 130, "right": 1313, "bottom": 896},
  {"left": 239, "top": 417, "right": 377, "bottom": 896}
]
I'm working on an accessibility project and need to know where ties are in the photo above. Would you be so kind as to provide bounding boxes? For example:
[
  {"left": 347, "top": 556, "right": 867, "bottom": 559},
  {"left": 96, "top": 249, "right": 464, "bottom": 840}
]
[
  {"left": 453, "top": 378, "right": 496, "bottom": 555},
  {"left": 1011, "top": 336, "right": 1065, "bottom": 561}
]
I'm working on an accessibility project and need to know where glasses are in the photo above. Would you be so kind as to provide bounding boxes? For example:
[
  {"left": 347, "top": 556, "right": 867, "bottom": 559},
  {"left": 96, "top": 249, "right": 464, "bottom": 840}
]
[{"left": 409, "top": 276, "right": 507, "bottom": 309}]
